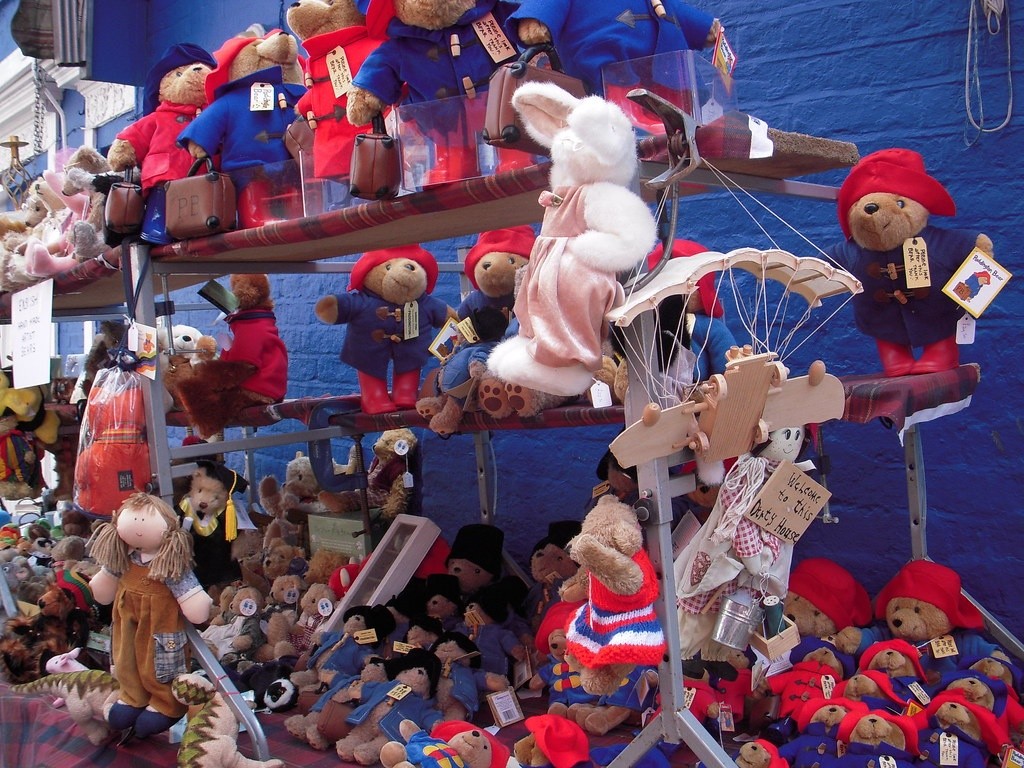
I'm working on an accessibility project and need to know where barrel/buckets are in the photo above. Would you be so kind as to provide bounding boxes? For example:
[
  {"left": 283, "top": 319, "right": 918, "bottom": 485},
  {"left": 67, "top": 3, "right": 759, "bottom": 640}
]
[{"left": 710, "top": 580, "right": 765, "bottom": 652}]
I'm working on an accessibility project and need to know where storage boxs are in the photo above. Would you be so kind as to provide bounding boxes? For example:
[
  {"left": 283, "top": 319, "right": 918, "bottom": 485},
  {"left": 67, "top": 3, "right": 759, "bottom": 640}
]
[
  {"left": 602, "top": 49, "right": 738, "bottom": 141},
  {"left": 306, "top": 509, "right": 386, "bottom": 567},
  {"left": 299, "top": 143, "right": 374, "bottom": 219},
  {"left": 750, "top": 613, "right": 800, "bottom": 660},
  {"left": 395, "top": 92, "right": 535, "bottom": 193},
  {"left": 230, "top": 156, "right": 299, "bottom": 228}
]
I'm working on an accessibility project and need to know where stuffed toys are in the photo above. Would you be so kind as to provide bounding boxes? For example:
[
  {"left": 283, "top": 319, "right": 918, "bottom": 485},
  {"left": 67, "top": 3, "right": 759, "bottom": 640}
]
[
  {"left": 283, "top": 520, "right": 1024, "bottom": 767},
  {"left": 259, "top": 452, "right": 330, "bottom": 520},
  {"left": 558, "top": 444, "right": 667, "bottom": 694},
  {"left": 1, "top": 0, "right": 723, "bottom": 291},
  {"left": 314, "top": 224, "right": 537, "bottom": 414},
  {"left": 585, "top": 449, "right": 640, "bottom": 514},
  {"left": 1, "top": 274, "right": 288, "bottom": 767},
  {"left": 815, "top": 146, "right": 994, "bottom": 375},
  {"left": 485, "top": 82, "right": 658, "bottom": 396},
  {"left": 649, "top": 240, "right": 739, "bottom": 483},
  {"left": 318, "top": 428, "right": 418, "bottom": 520},
  {"left": 676, "top": 422, "right": 819, "bottom": 675}
]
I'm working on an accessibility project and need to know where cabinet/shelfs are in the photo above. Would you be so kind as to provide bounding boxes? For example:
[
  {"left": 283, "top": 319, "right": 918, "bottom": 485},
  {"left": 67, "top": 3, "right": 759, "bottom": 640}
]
[{"left": 0, "top": 112, "right": 1024, "bottom": 768}]
